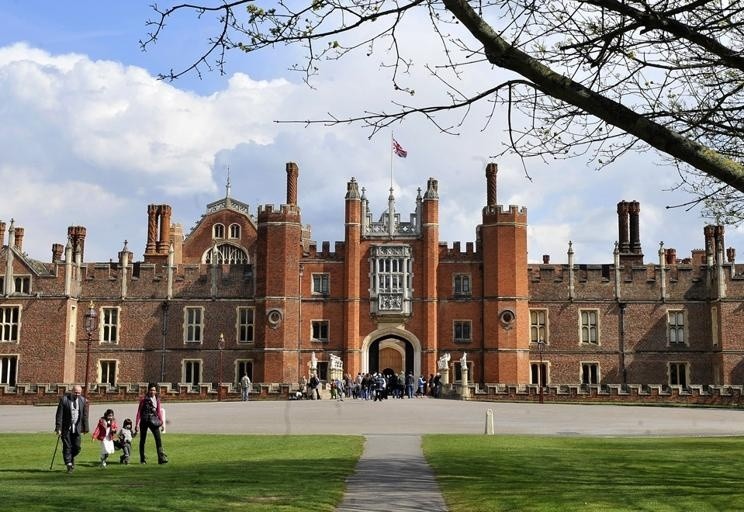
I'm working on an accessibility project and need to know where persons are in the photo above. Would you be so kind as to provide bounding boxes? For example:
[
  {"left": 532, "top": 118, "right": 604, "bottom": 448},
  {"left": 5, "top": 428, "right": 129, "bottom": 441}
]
[
  {"left": 287, "top": 370, "right": 443, "bottom": 401},
  {"left": 119, "top": 418, "right": 137, "bottom": 465},
  {"left": 55, "top": 384, "right": 89, "bottom": 471},
  {"left": 241, "top": 373, "right": 251, "bottom": 401},
  {"left": 135, "top": 382, "right": 169, "bottom": 464},
  {"left": 92, "top": 409, "right": 118, "bottom": 467}
]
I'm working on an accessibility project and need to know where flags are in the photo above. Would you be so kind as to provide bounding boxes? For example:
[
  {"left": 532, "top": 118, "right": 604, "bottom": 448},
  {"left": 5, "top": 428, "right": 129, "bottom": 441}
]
[{"left": 392, "top": 136, "right": 407, "bottom": 157}]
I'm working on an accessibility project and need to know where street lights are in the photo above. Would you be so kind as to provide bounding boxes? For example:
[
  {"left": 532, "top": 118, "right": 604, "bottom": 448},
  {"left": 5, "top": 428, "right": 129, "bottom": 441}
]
[
  {"left": 83, "top": 300, "right": 100, "bottom": 400},
  {"left": 538, "top": 335, "right": 545, "bottom": 404},
  {"left": 217, "top": 333, "right": 225, "bottom": 402}
]
